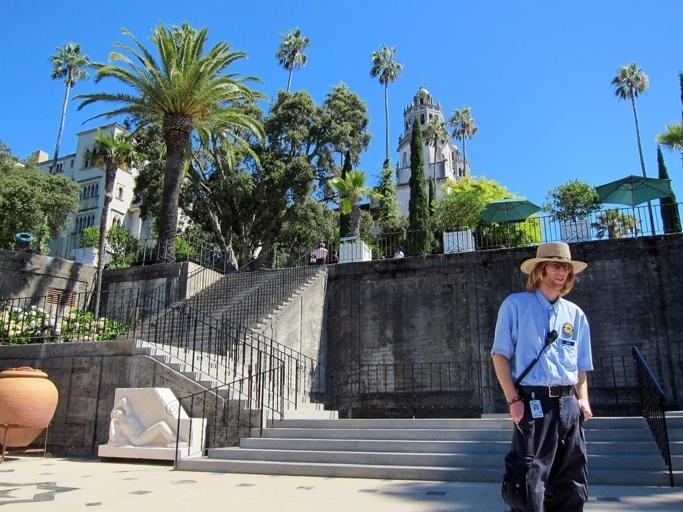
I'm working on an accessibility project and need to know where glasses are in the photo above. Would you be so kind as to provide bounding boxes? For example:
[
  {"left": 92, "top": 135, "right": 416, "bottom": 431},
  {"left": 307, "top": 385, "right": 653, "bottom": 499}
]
[{"left": 546, "top": 263, "right": 568, "bottom": 270}]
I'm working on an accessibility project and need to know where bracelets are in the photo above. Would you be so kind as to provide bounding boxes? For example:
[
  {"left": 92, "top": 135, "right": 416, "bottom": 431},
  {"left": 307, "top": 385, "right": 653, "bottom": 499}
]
[{"left": 507, "top": 396, "right": 522, "bottom": 406}]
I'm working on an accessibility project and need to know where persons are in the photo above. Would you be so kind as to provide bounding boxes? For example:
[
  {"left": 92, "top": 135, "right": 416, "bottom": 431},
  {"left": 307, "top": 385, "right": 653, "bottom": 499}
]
[
  {"left": 393, "top": 246, "right": 404, "bottom": 259},
  {"left": 488, "top": 242, "right": 595, "bottom": 511},
  {"left": 328, "top": 248, "right": 338, "bottom": 263},
  {"left": 314, "top": 243, "right": 328, "bottom": 264}
]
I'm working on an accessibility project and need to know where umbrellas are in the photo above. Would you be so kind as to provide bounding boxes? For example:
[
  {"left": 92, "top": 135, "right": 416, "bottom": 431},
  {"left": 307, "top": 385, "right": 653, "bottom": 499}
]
[
  {"left": 481, "top": 197, "right": 542, "bottom": 246},
  {"left": 594, "top": 173, "right": 673, "bottom": 237}
]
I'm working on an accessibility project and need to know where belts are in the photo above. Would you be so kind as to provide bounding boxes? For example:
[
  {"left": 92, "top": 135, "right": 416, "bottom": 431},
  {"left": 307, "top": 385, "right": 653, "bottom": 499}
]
[{"left": 519, "top": 385, "right": 574, "bottom": 397}]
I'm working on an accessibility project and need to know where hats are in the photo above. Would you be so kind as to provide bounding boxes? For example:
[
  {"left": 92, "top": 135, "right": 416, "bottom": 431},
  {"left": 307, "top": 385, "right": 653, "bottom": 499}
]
[{"left": 520, "top": 243, "right": 588, "bottom": 275}]
